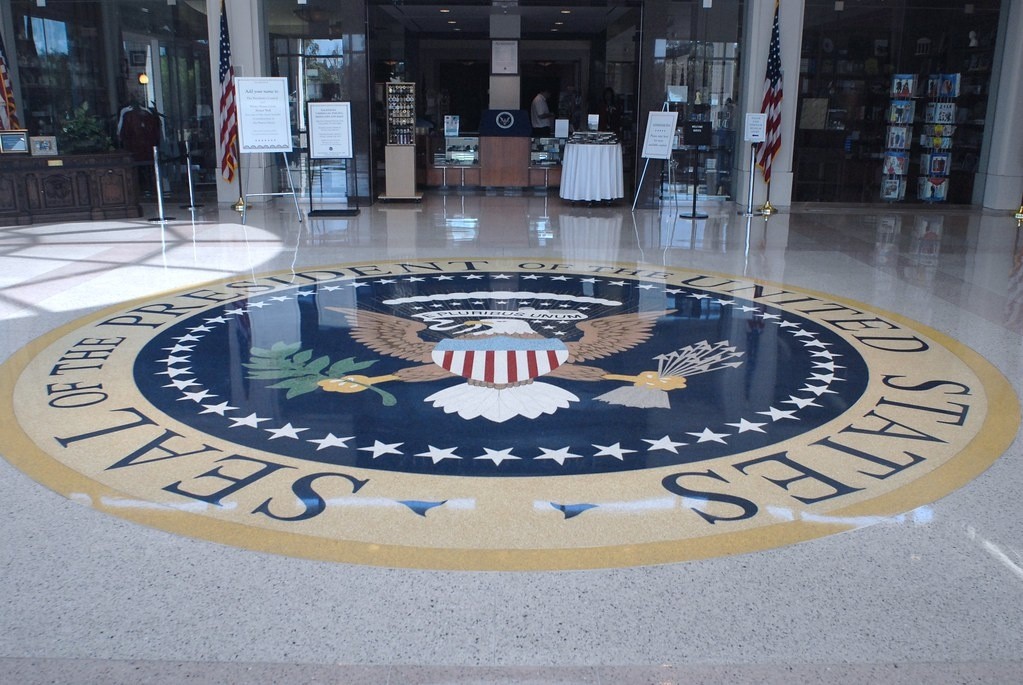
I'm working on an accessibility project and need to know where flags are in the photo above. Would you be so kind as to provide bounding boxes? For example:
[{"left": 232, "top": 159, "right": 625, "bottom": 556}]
[
  {"left": 218, "top": 0, "right": 239, "bottom": 183},
  {"left": 0, "top": 34, "right": 21, "bottom": 131},
  {"left": 754, "top": 0, "right": 782, "bottom": 184}
]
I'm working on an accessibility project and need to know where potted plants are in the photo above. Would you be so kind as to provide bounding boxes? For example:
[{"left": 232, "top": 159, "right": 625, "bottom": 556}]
[{"left": 63, "top": 112, "right": 117, "bottom": 155}]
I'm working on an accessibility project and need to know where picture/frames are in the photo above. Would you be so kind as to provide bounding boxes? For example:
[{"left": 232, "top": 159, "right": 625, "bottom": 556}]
[
  {"left": 0, "top": 128, "right": 31, "bottom": 156},
  {"left": 28, "top": 133, "right": 61, "bottom": 158}
]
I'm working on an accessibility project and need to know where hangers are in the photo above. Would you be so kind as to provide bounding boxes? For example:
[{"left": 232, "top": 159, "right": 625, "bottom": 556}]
[{"left": 117, "top": 99, "right": 159, "bottom": 123}]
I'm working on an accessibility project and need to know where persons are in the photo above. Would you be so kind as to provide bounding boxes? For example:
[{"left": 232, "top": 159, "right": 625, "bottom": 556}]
[
  {"left": 596, "top": 87, "right": 621, "bottom": 140},
  {"left": 530, "top": 85, "right": 555, "bottom": 138}
]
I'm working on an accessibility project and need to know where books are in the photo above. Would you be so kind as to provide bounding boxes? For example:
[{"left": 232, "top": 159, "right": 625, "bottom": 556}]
[{"left": 880, "top": 71, "right": 961, "bottom": 202}]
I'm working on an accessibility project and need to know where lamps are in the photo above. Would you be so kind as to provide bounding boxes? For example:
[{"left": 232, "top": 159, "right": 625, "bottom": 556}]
[
  {"left": 965, "top": 4, "right": 975, "bottom": 15},
  {"left": 36, "top": 0, "right": 47, "bottom": 8},
  {"left": 703, "top": 0, "right": 713, "bottom": 8},
  {"left": 297, "top": 0, "right": 308, "bottom": 5},
  {"left": 167, "top": 0, "right": 176, "bottom": 6},
  {"left": 833, "top": 1, "right": 845, "bottom": 12}
]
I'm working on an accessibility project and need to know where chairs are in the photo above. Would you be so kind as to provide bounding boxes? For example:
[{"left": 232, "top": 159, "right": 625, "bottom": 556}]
[
  {"left": 434, "top": 136, "right": 477, "bottom": 190},
  {"left": 529, "top": 136, "right": 560, "bottom": 191}
]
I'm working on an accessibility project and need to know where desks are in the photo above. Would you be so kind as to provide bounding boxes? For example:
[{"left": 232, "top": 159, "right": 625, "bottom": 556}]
[
  {"left": 0, "top": 147, "right": 145, "bottom": 227},
  {"left": 559, "top": 143, "right": 625, "bottom": 208}
]
